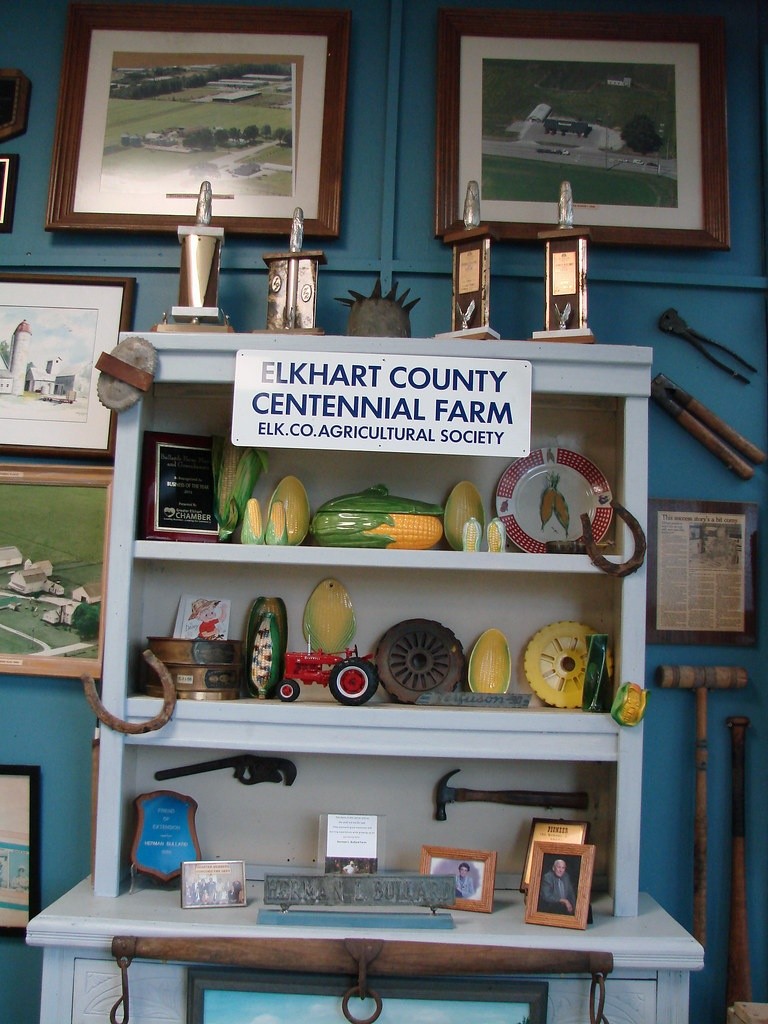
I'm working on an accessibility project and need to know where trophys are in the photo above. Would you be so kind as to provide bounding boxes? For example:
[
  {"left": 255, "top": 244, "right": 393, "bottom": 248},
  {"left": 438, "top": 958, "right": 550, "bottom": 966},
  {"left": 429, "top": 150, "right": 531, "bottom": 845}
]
[
  {"left": 251, "top": 206, "right": 327, "bottom": 335},
  {"left": 529, "top": 179, "right": 595, "bottom": 344},
  {"left": 434, "top": 181, "right": 502, "bottom": 341},
  {"left": 152, "top": 180, "right": 238, "bottom": 335}
]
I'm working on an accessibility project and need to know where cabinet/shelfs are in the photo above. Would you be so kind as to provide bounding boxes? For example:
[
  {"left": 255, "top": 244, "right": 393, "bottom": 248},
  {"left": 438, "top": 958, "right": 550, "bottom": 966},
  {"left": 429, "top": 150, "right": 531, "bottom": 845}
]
[{"left": 25, "top": 326, "right": 703, "bottom": 1023}]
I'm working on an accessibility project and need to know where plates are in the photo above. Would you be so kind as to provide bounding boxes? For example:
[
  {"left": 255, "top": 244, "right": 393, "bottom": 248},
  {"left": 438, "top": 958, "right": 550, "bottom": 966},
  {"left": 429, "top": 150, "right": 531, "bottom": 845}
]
[
  {"left": 267, "top": 475, "right": 310, "bottom": 546},
  {"left": 468, "top": 629, "right": 512, "bottom": 694},
  {"left": 495, "top": 447, "right": 614, "bottom": 554},
  {"left": 444, "top": 481, "right": 486, "bottom": 551},
  {"left": 303, "top": 579, "right": 356, "bottom": 660}
]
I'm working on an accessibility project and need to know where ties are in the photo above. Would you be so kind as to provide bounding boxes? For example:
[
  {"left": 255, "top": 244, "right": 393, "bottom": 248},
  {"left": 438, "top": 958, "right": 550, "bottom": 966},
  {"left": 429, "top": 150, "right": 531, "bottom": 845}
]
[{"left": 558, "top": 879, "right": 564, "bottom": 898}]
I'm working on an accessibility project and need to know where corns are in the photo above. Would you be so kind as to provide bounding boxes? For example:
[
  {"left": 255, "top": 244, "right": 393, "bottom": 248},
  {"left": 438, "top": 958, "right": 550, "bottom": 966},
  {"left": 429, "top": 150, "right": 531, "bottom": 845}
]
[
  {"left": 211, "top": 425, "right": 507, "bottom": 553},
  {"left": 242, "top": 579, "right": 511, "bottom": 700},
  {"left": 611, "top": 682, "right": 649, "bottom": 727}
]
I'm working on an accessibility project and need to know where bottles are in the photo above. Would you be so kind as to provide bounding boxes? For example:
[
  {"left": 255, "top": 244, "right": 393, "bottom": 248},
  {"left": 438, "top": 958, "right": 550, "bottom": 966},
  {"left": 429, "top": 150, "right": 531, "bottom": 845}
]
[{"left": 582, "top": 633, "right": 609, "bottom": 712}]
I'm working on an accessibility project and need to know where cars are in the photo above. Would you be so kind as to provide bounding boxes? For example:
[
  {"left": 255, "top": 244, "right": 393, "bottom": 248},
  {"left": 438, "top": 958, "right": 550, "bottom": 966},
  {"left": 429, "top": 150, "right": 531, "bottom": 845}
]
[
  {"left": 633, "top": 160, "right": 643, "bottom": 164},
  {"left": 537, "top": 148, "right": 569, "bottom": 155},
  {"left": 35, "top": 388, "right": 42, "bottom": 393}
]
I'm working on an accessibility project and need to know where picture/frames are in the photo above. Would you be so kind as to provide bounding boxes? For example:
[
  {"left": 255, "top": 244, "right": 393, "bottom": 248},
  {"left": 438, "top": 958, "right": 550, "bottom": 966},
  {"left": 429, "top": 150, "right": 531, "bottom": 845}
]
[
  {"left": 0, "top": 463, "right": 113, "bottom": 679},
  {"left": 524, "top": 839, "right": 596, "bottom": 929},
  {"left": 434, "top": 3, "right": 731, "bottom": 251},
  {"left": 0, "top": 271, "right": 135, "bottom": 459},
  {"left": 0, "top": 762, "right": 42, "bottom": 937},
  {"left": 180, "top": 858, "right": 248, "bottom": 909},
  {"left": 420, "top": 843, "right": 497, "bottom": 912},
  {"left": 43, "top": 1, "right": 350, "bottom": 236}
]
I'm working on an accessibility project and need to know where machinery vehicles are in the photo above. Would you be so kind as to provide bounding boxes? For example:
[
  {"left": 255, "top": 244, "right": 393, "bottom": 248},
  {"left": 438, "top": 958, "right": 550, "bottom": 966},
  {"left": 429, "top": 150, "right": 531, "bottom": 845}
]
[{"left": 276, "top": 634, "right": 379, "bottom": 705}]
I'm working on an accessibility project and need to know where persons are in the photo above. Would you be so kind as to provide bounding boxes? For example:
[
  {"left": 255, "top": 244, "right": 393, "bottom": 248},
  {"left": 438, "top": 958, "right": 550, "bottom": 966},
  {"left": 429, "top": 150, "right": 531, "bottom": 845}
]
[
  {"left": 11, "top": 864, "right": 29, "bottom": 891},
  {"left": 186, "top": 876, "right": 242, "bottom": 904},
  {"left": 0, "top": 853, "right": 9, "bottom": 888},
  {"left": 539, "top": 859, "right": 575, "bottom": 916},
  {"left": 455, "top": 863, "right": 475, "bottom": 898},
  {"left": 334, "top": 861, "right": 358, "bottom": 874}
]
